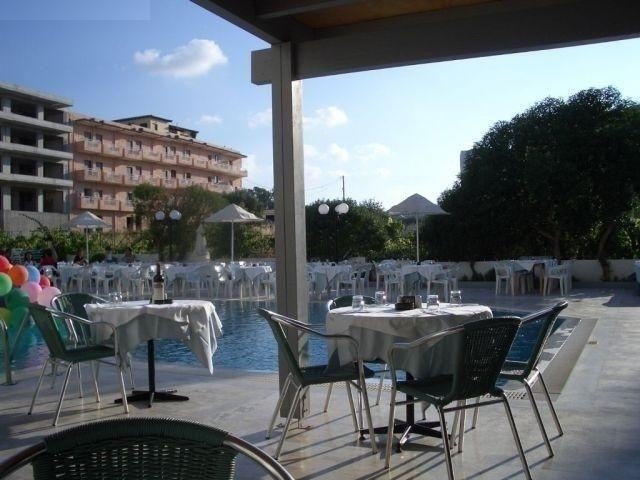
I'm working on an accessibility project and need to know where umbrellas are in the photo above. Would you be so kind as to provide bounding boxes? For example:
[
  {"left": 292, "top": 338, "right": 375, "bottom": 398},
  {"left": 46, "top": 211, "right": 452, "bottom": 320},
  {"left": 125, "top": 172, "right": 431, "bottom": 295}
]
[
  {"left": 203, "top": 202, "right": 265, "bottom": 262},
  {"left": 62, "top": 210, "right": 110, "bottom": 268},
  {"left": 385, "top": 193, "right": 451, "bottom": 264}
]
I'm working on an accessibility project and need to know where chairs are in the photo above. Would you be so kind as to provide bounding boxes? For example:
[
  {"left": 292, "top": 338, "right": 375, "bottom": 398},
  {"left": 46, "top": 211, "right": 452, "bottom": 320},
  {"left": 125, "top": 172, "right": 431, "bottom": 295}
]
[
  {"left": 450, "top": 301, "right": 569, "bottom": 459},
  {"left": 38, "top": 258, "right": 276, "bottom": 303},
  {"left": 22, "top": 303, "right": 130, "bottom": 426},
  {"left": 307, "top": 258, "right": 462, "bottom": 305},
  {"left": 491, "top": 258, "right": 573, "bottom": 297},
  {"left": 323, "top": 294, "right": 426, "bottom": 428},
  {"left": 256, "top": 307, "right": 377, "bottom": 460},
  {"left": 0, "top": 418, "right": 294, "bottom": 480},
  {"left": 384, "top": 316, "right": 533, "bottom": 480},
  {"left": 49, "top": 292, "right": 135, "bottom": 398}
]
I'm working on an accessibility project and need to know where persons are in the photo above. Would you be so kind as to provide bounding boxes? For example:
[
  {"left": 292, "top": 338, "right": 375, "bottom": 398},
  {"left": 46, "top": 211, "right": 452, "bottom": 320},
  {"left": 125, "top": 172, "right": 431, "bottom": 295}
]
[
  {"left": 72, "top": 248, "right": 87, "bottom": 267},
  {"left": 40, "top": 248, "right": 57, "bottom": 276},
  {"left": 24, "top": 252, "right": 34, "bottom": 266},
  {"left": 123, "top": 248, "right": 134, "bottom": 261},
  {"left": 72, "top": 259, "right": 85, "bottom": 278}
]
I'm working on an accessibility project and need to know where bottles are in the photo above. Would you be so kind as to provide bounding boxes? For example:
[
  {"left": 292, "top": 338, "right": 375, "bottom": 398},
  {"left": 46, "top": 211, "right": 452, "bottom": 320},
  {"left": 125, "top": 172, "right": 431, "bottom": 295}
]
[{"left": 152, "top": 263, "right": 165, "bottom": 305}]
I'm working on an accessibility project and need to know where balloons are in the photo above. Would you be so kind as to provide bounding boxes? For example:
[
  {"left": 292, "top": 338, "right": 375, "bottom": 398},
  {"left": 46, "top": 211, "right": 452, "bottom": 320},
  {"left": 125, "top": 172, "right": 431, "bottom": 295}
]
[{"left": 0, "top": 255, "right": 63, "bottom": 330}]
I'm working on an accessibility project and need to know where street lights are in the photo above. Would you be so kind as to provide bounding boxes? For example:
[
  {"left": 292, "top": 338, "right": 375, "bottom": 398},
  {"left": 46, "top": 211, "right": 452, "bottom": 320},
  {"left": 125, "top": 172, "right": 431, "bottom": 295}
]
[
  {"left": 155, "top": 208, "right": 183, "bottom": 263},
  {"left": 318, "top": 201, "right": 351, "bottom": 263}
]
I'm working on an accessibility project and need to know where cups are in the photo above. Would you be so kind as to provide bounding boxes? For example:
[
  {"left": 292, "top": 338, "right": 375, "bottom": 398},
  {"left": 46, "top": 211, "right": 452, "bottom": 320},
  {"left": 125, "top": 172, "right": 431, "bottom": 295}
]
[
  {"left": 426, "top": 295, "right": 440, "bottom": 311},
  {"left": 414, "top": 295, "right": 422, "bottom": 308},
  {"left": 352, "top": 295, "right": 364, "bottom": 311},
  {"left": 450, "top": 290, "right": 463, "bottom": 306},
  {"left": 108, "top": 291, "right": 122, "bottom": 307},
  {"left": 375, "top": 290, "right": 386, "bottom": 305}
]
